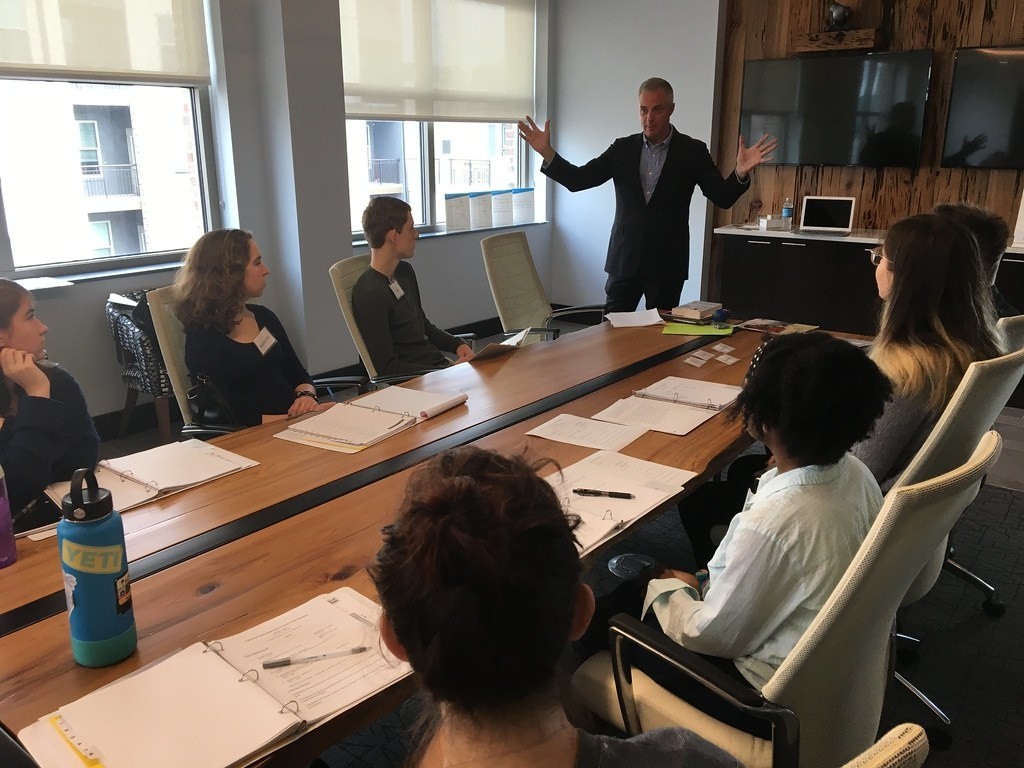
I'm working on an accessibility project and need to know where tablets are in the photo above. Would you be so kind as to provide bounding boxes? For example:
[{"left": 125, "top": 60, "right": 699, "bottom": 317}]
[{"left": 800, "top": 196, "right": 856, "bottom": 234}]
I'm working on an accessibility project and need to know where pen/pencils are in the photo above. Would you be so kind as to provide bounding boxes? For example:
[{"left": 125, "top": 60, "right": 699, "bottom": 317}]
[
  {"left": 572, "top": 487, "right": 635, "bottom": 499},
  {"left": 263, "top": 645, "right": 372, "bottom": 670},
  {"left": 327, "top": 387, "right": 339, "bottom": 403},
  {"left": 12, "top": 495, "right": 40, "bottom": 524},
  {"left": 445, "top": 356, "right": 454, "bottom": 363}
]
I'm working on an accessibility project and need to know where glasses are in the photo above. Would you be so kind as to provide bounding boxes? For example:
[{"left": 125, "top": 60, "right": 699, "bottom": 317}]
[{"left": 864, "top": 244, "right": 895, "bottom": 267}]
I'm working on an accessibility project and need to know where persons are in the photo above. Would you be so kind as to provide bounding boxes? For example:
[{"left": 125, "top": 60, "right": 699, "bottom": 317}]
[
  {"left": 518, "top": 77, "right": 777, "bottom": 322},
  {"left": 351, "top": 196, "right": 474, "bottom": 372},
  {"left": 0, "top": 278, "right": 99, "bottom": 506},
  {"left": 371, "top": 444, "right": 742, "bottom": 768},
  {"left": 679, "top": 201, "right": 1023, "bottom": 570},
  {"left": 580, "top": 332, "right": 895, "bottom": 694},
  {"left": 175, "top": 229, "right": 337, "bottom": 429}
]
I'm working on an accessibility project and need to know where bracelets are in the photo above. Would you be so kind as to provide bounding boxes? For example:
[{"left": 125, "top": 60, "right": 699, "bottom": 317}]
[
  {"left": 294, "top": 391, "right": 318, "bottom": 400},
  {"left": 696, "top": 569, "right": 708, "bottom": 594}
]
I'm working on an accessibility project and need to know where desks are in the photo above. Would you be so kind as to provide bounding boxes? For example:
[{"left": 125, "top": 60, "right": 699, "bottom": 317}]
[{"left": 0, "top": 310, "right": 879, "bottom": 768}]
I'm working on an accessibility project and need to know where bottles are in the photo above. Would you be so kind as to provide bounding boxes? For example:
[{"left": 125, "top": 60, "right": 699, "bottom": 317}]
[
  {"left": 0, "top": 464, "right": 16, "bottom": 570},
  {"left": 57, "top": 469, "right": 138, "bottom": 668},
  {"left": 781, "top": 198, "right": 793, "bottom": 231}
]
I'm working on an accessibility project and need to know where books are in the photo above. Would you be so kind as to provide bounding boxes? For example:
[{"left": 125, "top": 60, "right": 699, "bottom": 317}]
[
  {"left": 662, "top": 322, "right": 734, "bottom": 335},
  {"left": 591, "top": 376, "right": 743, "bottom": 434},
  {"left": 468, "top": 327, "right": 532, "bottom": 360},
  {"left": 19, "top": 585, "right": 413, "bottom": 768},
  {"left": 272, "top": 383, "right": 468, "bottom": 455},
  {"left": 542, "top": 450, "right": 697, "bottom": 558},
  {"left": 43, "top": 438, "right": 261, "bottom": 513}
]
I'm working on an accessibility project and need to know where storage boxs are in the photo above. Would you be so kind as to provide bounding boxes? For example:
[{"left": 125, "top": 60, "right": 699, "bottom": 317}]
[{"left": 760, "top": 219, "right": 783, "bottom": 230}]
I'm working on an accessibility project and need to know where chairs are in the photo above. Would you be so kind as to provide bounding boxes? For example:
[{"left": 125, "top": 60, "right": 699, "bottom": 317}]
[
  {"left": 565, "top": 430, "right": 1003, "bottom": 768},
  {"left": 329, "top": 252, "right": 477, "bottom": 386},
  {"left": 481, "top": 231, "right": 609, "bottom": 346},
  {"left": 847, "top": 314, "right": 1024, "bottom": 643},
  {"left": 106, "top": 287, "right": 173, "bottom": 446},
  {"left": 146, "top": 286, "right": 372, "bottom": 439}
]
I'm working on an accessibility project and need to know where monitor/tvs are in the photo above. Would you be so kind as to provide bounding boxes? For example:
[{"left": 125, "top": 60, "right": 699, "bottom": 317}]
[
  {"left": 939, "top": 46, "right": 1024, "bottom": 167},
  {"left": 739, "top": 48, "right": 934, "bottom": 167}
]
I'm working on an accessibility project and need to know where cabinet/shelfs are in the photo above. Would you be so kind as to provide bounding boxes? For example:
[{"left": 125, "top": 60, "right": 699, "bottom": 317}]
[{"left": 714, "top": 223, "right": 1024, "bottom": 409}]
